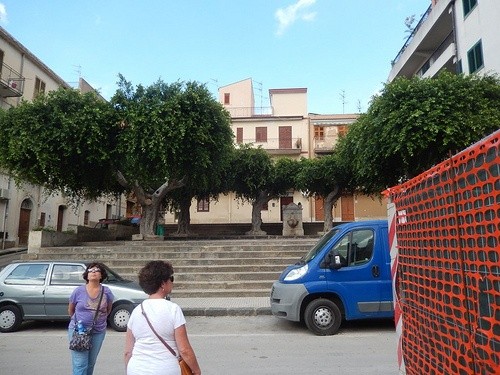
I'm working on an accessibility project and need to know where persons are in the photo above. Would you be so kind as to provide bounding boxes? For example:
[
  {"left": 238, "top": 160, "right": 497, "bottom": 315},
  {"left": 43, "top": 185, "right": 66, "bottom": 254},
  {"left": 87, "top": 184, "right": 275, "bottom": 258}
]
[
  {"left": 68, "top": 263, "right": 114, "bottom": 375},
  {"left": 124, "top": 260, "right": 202, "bottom": 375}
]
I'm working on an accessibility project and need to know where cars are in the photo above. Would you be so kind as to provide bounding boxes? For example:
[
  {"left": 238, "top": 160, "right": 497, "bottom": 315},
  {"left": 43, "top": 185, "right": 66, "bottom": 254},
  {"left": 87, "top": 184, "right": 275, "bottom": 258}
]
[{"left": 0, "top": 261, "right": 171, "bottom": 332}]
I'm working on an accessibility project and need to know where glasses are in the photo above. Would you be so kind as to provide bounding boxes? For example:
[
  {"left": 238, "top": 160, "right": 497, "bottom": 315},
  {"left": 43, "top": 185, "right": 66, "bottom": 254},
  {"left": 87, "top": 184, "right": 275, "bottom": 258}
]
[
  {"left": 169, "top": 276, "right": 174, "bottom": 282},
  {"left": 87, "top": 269, "right": 101, "bottom": 273}
]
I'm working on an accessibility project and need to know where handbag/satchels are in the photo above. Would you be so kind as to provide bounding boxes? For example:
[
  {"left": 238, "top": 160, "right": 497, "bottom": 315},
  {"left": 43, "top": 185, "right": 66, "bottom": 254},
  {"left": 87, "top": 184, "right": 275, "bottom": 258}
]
[
  {"left": 177, "top": 355, "right": 193, "bottom": 375},
  {"left": 69, "top": 323, "right": 95, "bottom": 352}
]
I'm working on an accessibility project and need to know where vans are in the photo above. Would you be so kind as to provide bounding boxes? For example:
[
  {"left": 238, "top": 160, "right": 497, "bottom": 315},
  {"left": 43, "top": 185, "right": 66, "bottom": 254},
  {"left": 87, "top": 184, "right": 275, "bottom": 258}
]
[{"left": 270, "top": 220, "right": 394, "bottom": 336}]
